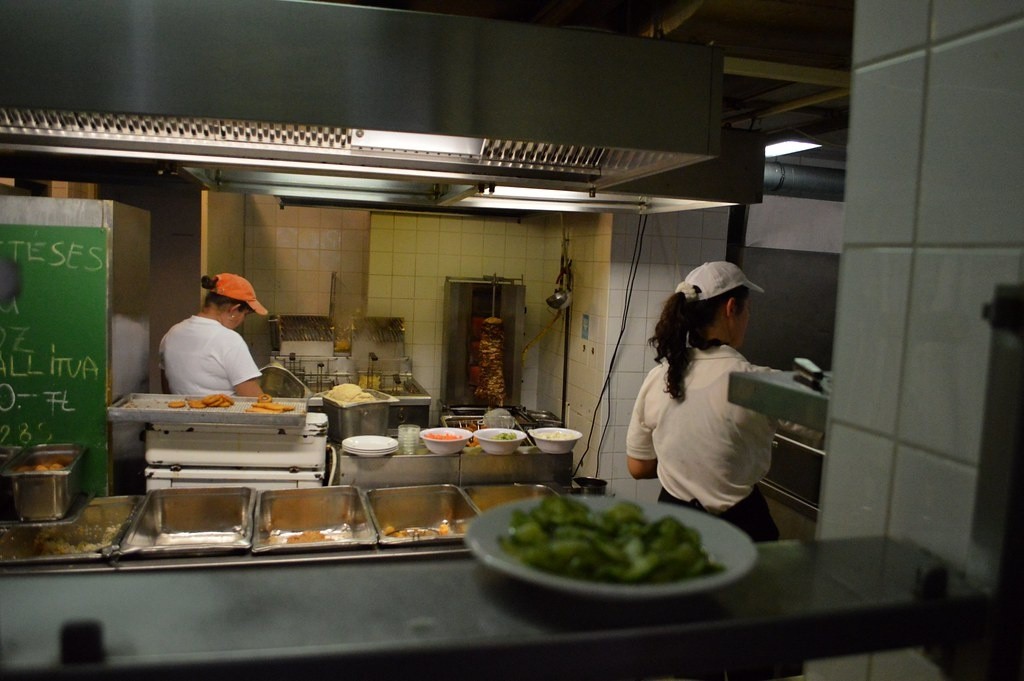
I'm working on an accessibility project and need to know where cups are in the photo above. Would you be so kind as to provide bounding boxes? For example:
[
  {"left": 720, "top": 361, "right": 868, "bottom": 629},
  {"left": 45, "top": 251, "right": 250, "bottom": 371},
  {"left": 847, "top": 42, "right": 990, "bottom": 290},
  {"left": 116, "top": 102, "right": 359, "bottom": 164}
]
[{"left": 399, "top": 425, "right": 420, "bottom": 454}]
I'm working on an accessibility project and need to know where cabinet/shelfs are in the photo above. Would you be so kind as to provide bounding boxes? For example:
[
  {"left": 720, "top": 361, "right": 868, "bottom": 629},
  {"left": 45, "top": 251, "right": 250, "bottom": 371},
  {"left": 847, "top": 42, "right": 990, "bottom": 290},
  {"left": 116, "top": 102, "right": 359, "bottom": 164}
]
[{"left": 0, "top": 535, "right": 1023, "bottom": 681}]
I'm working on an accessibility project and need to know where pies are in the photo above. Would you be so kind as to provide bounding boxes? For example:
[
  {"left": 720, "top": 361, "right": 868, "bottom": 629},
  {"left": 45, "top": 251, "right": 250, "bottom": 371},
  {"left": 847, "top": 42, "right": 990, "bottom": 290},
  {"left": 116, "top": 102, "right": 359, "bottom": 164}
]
[
  {"left": 245, "top": 402, "right": 294, "bottom": 413},
  {"left": 168, "top": 393, "right": 234, "bottom": 409}
]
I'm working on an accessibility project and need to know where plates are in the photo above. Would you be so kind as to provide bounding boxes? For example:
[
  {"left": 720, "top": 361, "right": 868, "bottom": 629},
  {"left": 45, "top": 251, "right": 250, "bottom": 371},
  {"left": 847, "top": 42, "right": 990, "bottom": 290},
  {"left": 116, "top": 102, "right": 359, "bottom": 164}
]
[
  {"left": 464, "top": 495, "right": 757, "bottom": 599},
  {"left": 342, "top": 435, "right": 399, "bottom": 457}
]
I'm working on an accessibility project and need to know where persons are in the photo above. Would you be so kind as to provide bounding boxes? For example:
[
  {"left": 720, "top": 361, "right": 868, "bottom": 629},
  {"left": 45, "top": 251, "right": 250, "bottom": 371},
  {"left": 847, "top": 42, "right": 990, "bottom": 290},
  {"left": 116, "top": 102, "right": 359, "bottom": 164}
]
[
  {"left": 158, "top": 273, "right": 269, "bottom": 398},
  {"left": 626, "top": 261, "right": 782, "bottom": 544}
]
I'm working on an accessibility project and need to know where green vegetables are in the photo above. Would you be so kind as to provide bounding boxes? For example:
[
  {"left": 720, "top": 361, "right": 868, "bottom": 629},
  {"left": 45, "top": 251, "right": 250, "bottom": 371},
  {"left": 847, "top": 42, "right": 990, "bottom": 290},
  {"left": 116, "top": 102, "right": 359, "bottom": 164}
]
[{"left": 498, "top": 493, "right": 720, "bottom": 581}]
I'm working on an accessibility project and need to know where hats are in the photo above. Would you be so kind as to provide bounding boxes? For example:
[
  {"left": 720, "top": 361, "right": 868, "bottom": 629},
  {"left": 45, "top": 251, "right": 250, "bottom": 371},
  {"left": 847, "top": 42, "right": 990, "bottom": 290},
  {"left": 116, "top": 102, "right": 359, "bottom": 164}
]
[
  {"left": 686, "top": 261, "right": 765, "bottom": 301},
  {"left": 209, "top": 273, "right": 269, "bottom": 316}
]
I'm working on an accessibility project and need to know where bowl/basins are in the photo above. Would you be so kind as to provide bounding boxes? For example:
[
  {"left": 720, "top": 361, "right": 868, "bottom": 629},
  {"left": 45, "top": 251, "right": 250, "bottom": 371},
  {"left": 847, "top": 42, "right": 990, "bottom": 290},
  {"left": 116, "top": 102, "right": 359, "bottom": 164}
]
[
  {"left": 459, "top": 484, "right": 561, "bottom": 510},
  {"left": 252, "top": 485, "right": 377, "bottom": 554},
  {"left": 1, "top": 446, "right": 23, "bottom": 471},
  {"left": 420, "top": 427, "right": 472, "bottom": 454},
  {"left": 1, "top": 495, "right": 144, "bottom": 565},
  {"left": 1, "top": 443, "right": 85, "bottom": 521},
  {"left": 119, "top": 487, "right": 255, "bottom": 559},
  {"left": 530, "top": 428, "right": 583, "bottom": 455},
  {"left": 313, "top": 388, "right": 399, "bottom": 444},
  {"left": 473, "top": 428, "right": 527, "bottom": 455},
  {"left": 364, "top": 482, "right": 480, "bottom": 555},
  {"left": 256, "top": 363, "right": 313, "bottom": 404}
]
